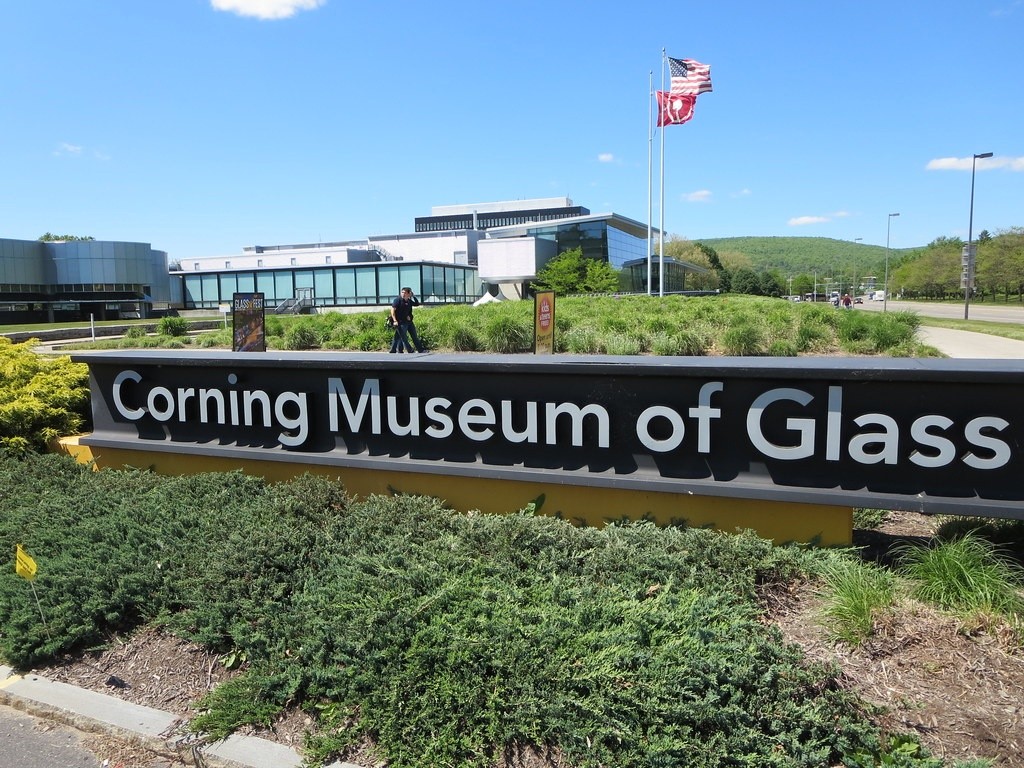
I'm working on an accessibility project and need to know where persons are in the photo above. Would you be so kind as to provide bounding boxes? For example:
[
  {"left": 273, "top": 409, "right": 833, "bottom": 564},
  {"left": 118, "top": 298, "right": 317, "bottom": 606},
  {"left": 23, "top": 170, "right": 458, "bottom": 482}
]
[
  {"left": 843, "top": 294, "right": 851, "bottom": 306},
  {"left": 390, "top": 287, "right": 429, "bottom": 353},
  {"left": 834, "top": 298, "right": 839, "bottom": 305}
]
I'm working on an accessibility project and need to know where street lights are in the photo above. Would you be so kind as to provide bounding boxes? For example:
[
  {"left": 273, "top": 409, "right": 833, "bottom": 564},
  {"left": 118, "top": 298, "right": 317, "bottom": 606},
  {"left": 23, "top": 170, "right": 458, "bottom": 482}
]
[
  {"left": 852, "top": 237, "right": 863, "bottom": 308},
  {"left": 964, "top": 151, "right": 994, "bottom": 319},
  {"left": 883, "top": 212, "right": 901, "bottom": 311}
]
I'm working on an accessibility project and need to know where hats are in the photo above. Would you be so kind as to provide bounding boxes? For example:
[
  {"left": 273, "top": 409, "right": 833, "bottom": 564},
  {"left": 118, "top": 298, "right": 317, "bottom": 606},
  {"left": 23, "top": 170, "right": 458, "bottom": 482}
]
[{"left": 845, "top": 294, "right": 848, "bottom": 296}]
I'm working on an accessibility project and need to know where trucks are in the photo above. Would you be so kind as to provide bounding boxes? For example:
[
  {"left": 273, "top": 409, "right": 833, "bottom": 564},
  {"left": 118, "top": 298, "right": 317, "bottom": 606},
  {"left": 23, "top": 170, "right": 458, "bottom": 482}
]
[{"left": 830, "top": 292, "right": 839, "bottom": 302}]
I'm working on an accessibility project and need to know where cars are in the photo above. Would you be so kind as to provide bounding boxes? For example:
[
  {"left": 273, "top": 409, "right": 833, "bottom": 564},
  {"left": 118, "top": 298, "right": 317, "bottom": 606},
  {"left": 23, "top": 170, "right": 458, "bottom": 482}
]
[{"left": 853, "top": 297, "right": 863, "bottom": 304}]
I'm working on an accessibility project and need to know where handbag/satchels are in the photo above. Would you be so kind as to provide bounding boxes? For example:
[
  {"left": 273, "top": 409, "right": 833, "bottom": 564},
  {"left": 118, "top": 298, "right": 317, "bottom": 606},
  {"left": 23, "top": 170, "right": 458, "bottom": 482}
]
[{"left": 384, "top": 316, "right": 398, "bottom": 329}]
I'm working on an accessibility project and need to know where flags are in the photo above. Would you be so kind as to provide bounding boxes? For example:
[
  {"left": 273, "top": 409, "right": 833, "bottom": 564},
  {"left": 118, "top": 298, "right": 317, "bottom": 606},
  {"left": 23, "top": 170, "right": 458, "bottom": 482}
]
[{"left": 656, "top": 55, "right": 712, "bottom": 127}]
